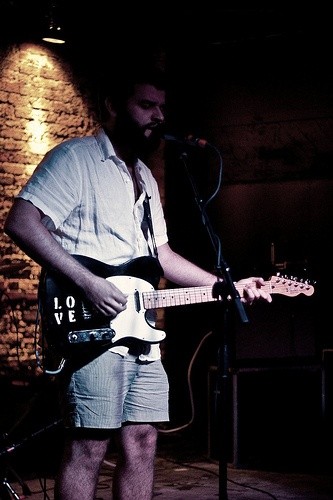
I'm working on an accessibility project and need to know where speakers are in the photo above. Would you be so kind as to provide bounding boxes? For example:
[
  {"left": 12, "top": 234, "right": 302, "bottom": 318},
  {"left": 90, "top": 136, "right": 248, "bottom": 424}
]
[{"left": 207, "top": 358, "right": 326, "bottom": 469}]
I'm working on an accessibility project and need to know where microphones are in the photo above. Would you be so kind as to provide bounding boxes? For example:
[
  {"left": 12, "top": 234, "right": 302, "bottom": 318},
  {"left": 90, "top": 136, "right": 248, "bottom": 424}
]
[{"left": 155, "top": 120, "right": 208, "bottom": 147}]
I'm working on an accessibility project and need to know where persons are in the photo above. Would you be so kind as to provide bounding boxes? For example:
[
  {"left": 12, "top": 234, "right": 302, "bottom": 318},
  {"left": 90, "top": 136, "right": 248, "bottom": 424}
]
[{"left": 4, "top": 75, "right": 273, "bottom": 499}]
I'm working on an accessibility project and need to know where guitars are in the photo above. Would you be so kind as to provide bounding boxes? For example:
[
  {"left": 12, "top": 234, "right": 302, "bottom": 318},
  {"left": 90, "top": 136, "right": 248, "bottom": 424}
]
[{"left": 39, "top": 255, "right": 316, "bottom": 355}]
